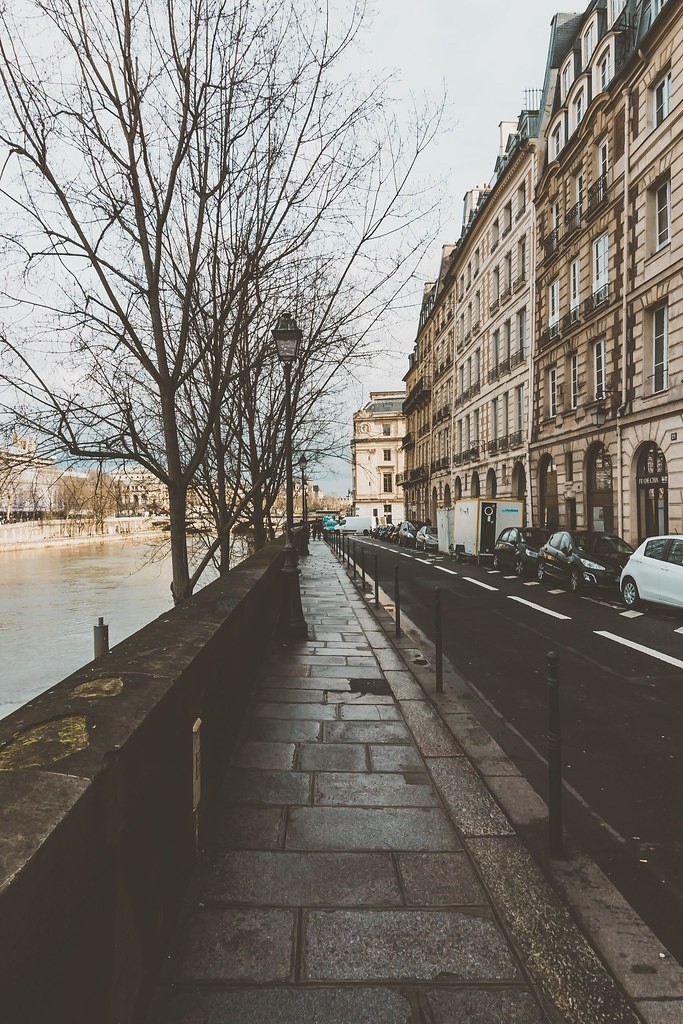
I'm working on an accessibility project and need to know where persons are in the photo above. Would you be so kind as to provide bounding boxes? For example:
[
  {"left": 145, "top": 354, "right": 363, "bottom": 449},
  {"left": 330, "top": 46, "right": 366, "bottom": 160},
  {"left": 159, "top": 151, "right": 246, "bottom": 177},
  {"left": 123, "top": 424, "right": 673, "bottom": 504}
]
[
  {"left": 311, "top": 523, "right": 322, "bottom": 540},
  {"left": 426, "top": 518, "right": 431, "bottom": 526}
]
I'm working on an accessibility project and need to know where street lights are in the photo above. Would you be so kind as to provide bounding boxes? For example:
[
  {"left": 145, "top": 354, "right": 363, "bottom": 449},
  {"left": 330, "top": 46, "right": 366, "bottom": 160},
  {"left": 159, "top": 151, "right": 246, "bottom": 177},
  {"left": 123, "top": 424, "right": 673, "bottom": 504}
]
[
  {"left": 298, "top": 453, "right": 308, "bottom": 526},
  {"left": 270, "top": 314, "right": 307, "bottom": 635}
]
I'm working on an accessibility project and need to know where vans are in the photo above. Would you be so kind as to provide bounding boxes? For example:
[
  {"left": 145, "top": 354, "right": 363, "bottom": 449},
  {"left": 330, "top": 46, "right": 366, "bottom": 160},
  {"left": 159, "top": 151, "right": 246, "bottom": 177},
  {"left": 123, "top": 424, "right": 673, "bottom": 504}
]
[{"left": 334, "top": 517, "right": 375, "bottom": 536}]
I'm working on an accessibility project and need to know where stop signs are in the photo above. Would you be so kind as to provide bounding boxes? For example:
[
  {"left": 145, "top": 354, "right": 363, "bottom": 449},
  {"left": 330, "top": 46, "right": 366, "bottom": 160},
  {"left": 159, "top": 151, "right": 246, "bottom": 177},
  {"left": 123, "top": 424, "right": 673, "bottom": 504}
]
[{"left": 341, "top": 511, "right": 346, "bottom": 516}]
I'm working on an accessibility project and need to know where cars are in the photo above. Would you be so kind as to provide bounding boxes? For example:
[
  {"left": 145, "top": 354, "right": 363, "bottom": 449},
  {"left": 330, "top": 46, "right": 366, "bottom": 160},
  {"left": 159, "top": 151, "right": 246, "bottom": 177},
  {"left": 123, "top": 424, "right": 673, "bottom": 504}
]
[
  {"left": 538, "top": 530, "right": 636, "bottom": 591},
  {"left": 617, "top": 534, "right": 682, "bottom": 610},
  {"left": 416, "top": 526, "right": 438, "bottom": 551},
  {"left": 398, "top": 520, "right": 429, "bottom": 547},
  {"left": 374, "top": 525, "right": 401, "bottom": 544},
  {"left": 493, "top": 525, "right": 551, "bottom": 576}
]
